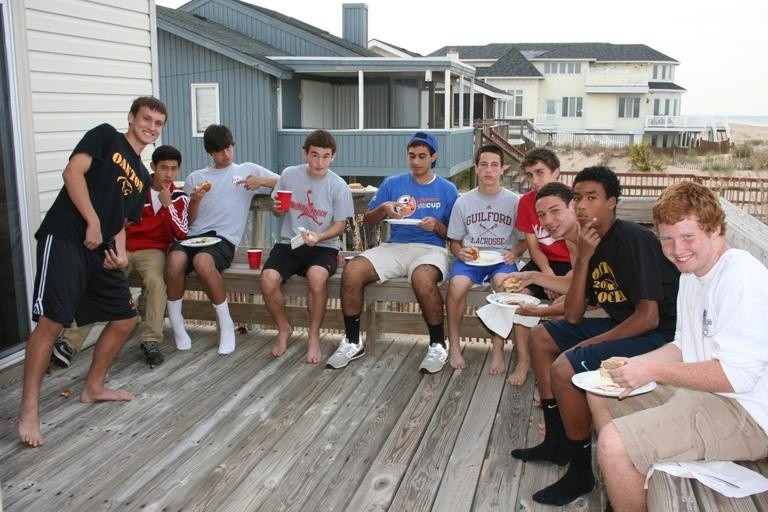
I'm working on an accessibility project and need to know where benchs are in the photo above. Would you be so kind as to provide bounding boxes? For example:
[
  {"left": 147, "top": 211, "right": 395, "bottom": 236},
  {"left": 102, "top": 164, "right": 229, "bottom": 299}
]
[{"left": 123, "top": 192, "right": 768, "bottom": 512}]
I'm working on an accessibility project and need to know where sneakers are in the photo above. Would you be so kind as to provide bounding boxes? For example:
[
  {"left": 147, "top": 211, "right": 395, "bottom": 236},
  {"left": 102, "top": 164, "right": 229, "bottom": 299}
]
[
  {"left": 326, "top": 336, "right": 364, "bottom": 369},
  {"left": 419, "top": 342, "right": 449, "bottom": 374},
  {"left": 141, "top": 340, "right": 164, "bottom": 366},
  {"left": 50, "top": 340, "right": 72, "bottom": 367}
]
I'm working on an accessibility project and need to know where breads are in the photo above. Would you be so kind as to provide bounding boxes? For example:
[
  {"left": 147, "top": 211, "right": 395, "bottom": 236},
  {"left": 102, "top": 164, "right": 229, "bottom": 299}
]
[
  {"left": 470, "top": 247, "right": 481, "bottom": 261},
  {"left": 195, "top": 180, "right": 211, "bottom": 192},
  {"left": 601, "top": 355, "right": 626, "bottom": 373},
  {"left": 396, "top": 196, "right": 416, "bottom": 218},
  {"left": 503, "top": 276, "right": 523, "bottom": 293},
  {"left": 351, "top": 182, "right": 364, "bottom": 189}
]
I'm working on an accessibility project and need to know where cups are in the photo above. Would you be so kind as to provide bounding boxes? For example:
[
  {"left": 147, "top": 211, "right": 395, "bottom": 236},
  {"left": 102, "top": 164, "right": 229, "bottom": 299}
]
[
  {"left": 345, "top": 256, "right": 353, "bottom": 264},
  {"left": 246, "top": 248, "right": 263, "bottom": 269},
  {"left": 276, "top": 190, "right": 292, "bottom": 212}
]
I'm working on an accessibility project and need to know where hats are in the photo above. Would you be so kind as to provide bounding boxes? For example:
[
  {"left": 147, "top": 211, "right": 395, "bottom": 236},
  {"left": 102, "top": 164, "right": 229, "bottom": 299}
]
[{"left": 408, "top": 133, "right": 437, "bottom": 168}]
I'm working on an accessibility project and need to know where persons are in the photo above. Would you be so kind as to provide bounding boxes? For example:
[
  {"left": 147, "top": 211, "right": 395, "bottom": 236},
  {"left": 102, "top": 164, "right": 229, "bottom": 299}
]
[
  {"left": 504, "top": 147, "right": 575, "bottom": 389},
  {"left": 50, "top": 144, "right": 190, "bottom": 369},
  {"left": 18, "top": 95, "right": 169, "bottom": 449},
  {"left": 442, "top": 145, "right": 527, "bottom": 376},
  {"left": 259, "top": 130, "right": 355, "bottom": 364},
  {"left": 497, "top": 182, "right": 583, "bottom": 437},
  {"left": 512, "top": 164, "right": 683, "bottom": 508},
  {"left": 584, "top": 181, "right": 766, "bottom": 512},
  {"left": 324, "top": 131, "right": 460, "bottom": 374},
  {"left": 166, "top": 123, "right": 281, "bottom": 358}
]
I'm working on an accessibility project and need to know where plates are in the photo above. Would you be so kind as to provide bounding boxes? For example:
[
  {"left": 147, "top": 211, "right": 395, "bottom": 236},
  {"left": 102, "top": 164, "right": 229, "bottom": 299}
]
[
  {"left": 485, "top": 293, "right": 541, "bottom": 309},
  {"left": 381, "top": 215, "right": 420, "bottom": 225},
  {"left": 573, "top": 371, "right": 656, "bottom": 397},
  {"left": 465, "top": 251, "right": 507, "bottom": 268},
  {"left": 180, "top": 236, "right": 224, "bottom": 248}
]
